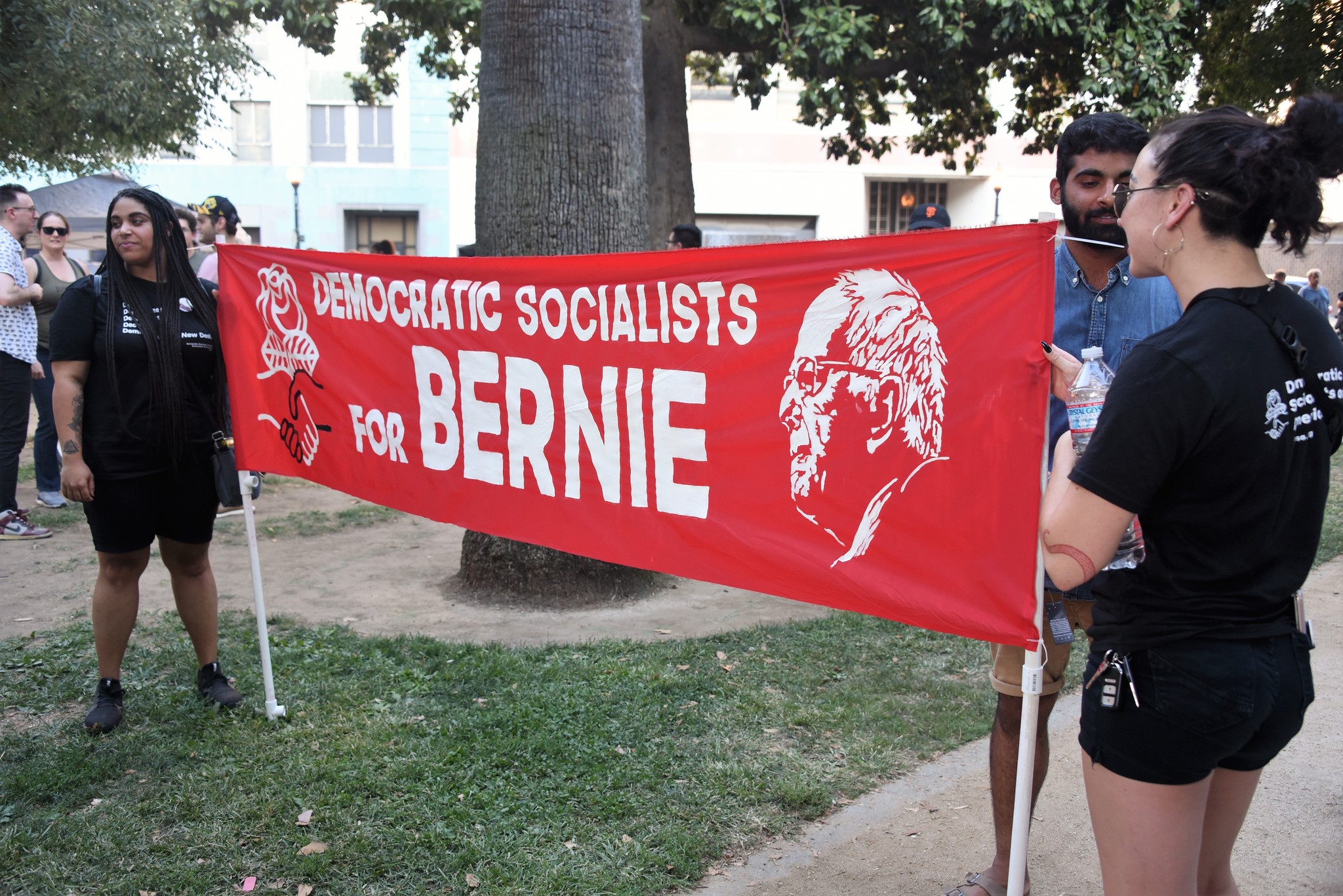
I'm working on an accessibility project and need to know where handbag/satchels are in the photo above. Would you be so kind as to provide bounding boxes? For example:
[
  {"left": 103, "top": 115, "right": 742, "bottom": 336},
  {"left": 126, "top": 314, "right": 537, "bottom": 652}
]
[{"left": 212, "top": 446, "right": 261, "bottom": 507}]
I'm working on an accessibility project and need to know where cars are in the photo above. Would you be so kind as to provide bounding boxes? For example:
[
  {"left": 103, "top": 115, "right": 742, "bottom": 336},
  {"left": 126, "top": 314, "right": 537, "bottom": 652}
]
[{"left": 1264, "top": 275, "right": 1310, "bottom": 294}]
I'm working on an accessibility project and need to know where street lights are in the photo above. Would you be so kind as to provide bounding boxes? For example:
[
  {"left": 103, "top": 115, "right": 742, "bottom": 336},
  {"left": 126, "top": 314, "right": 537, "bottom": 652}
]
[
  {"left": 994, "top": 186, "right": 1002, "bottom": 225},
  {"left": 290, "top": 172, "right": 301, "bottom": 250}
]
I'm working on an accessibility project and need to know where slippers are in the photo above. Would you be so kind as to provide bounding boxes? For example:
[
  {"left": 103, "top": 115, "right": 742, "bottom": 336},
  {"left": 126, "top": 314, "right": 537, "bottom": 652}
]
[{"left": 942, "top": 872, "right": 1031, "bottom": 896}]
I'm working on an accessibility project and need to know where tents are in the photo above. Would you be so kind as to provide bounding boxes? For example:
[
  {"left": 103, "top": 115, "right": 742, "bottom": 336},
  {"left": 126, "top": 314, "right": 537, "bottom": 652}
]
[{"left": 21, "top": 168, "right": 191, "bottom": 260}]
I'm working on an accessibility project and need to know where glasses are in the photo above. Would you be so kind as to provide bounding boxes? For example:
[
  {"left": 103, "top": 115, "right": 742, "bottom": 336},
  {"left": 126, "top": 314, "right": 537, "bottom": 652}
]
[
  {"left": 664, "top": 240, "right": 676, "bottom": 246},
  {"left": 1112, "top": 183, "right": 1211, "bottom": 217},
  {"left": 42, "top": 227, "right": 69, "bottom": 236},
  {"left": 4, "top": 207, "right": 36, "bottom": 213}
]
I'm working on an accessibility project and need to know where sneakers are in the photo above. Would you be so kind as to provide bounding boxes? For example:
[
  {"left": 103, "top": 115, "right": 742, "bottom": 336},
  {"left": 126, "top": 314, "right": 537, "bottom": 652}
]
[
  {"left": 14, "top": 508, "right": 32, "bottom": 517},
  {"left": 0, "top": 510, "right": 52, "bottom": 541},
  {"left": 197, "top": 661, "right": 242, "bottom": 710},
  {"left": 36, "top": 491, "right": 68, "bottom": 507},
  {"left": 84, "top": 677, "right": 125, "bottom": 733}
]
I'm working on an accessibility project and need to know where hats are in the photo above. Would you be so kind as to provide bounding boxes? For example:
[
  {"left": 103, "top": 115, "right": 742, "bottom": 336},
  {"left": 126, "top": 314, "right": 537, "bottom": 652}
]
[
  {"left": 188, "top": 196, "right": 241, "bottom": 224},
  {"left": 909, "top": 204, "right": 950, "bottom": 231}
]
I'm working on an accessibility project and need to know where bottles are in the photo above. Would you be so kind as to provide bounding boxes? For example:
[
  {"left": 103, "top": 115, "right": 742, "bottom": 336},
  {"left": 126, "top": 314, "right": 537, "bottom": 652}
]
[{"left": 1067, "top": 346, "right": 1145, "bottom": 572}]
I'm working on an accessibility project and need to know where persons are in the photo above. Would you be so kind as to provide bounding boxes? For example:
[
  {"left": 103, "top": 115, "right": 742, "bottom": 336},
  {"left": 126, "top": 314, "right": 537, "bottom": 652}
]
[
  {"left": 665, "top": 225, "right": 701, "bottom": 252},
  {"left": 23, "top": 212, "right": 90, "bottom": 508},
  {"left": 371, "top": 240, "right": 394, "bottom": 254},
  {"left": 1044, "top": 94, "right": 1343, "bottom": 896},
  {"left": 188, "top": 196, "right": 256, "bottom": 519},
  {"left": 1297, "top": 268, "right": 1329, "bottom": 321},
  {"left": 1335, "top": 292, "right": 1343, "bottom": 336},
  {"left": 0, "top": 183, "right": 53, "bottom": 542},
  {"left": 173, "top": 208, "right": 210, "bottom": 275},
  {"left": 49, "top": 187, "right": 242, "bottom": 735},
  {"left": 948, "top": 112, "right": 1181, "bottom": 895},
  {"left": 1272, "top": 269, "right": 1293, "bottom": 292}
]
